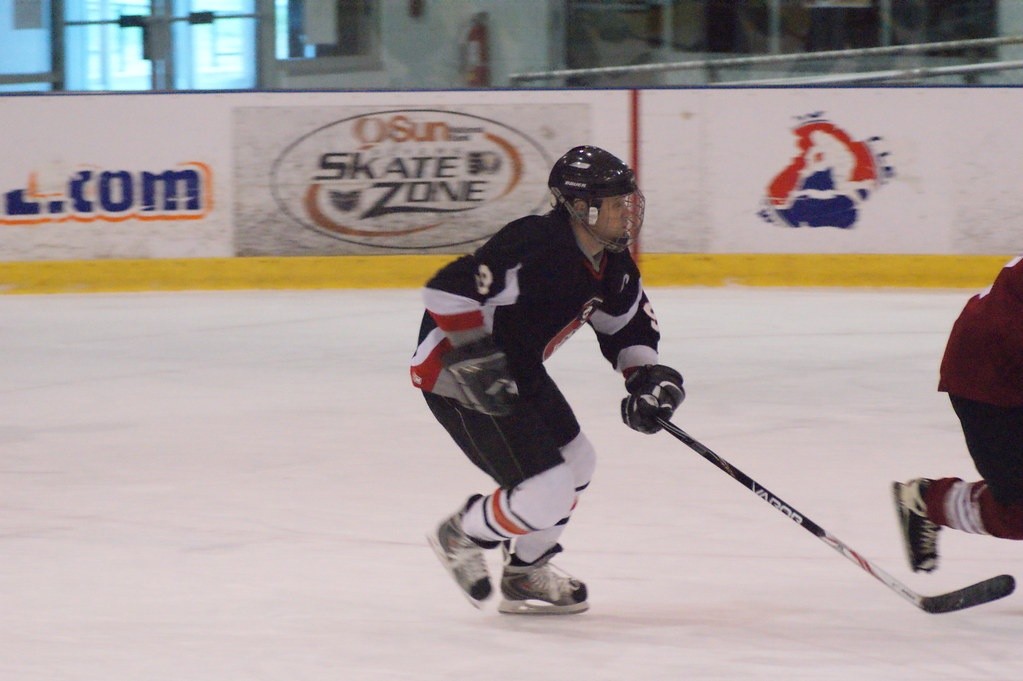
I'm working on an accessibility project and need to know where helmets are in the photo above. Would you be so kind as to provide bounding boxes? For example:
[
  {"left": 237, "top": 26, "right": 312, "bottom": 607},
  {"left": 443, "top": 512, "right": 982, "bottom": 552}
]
[{"left": 548, "top": 144, "right": 645, "bottom": 254}]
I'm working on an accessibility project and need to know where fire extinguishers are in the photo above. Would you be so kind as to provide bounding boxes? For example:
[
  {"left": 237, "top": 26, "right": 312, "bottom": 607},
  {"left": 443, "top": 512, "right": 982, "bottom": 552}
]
[{"left": 464, "top": 11, "right": 489, "bottom": 89}]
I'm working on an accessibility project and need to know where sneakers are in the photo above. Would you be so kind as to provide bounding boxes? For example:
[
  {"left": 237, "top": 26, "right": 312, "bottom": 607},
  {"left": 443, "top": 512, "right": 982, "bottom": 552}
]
[
  {"left": 428, "top": 493, "right": 500, "bottom": 611},
  {"left": 891, "top": 477, "right": 940, "bottom": 573},
  {"left": 497, "top": 539, "right": 590, "bottom": 614}
]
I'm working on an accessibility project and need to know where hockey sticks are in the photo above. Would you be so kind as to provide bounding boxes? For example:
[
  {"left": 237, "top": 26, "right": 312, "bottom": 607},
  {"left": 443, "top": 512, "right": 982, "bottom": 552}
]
[{"left": 650, "top": 414, "right": 1017, "bottom": 614}]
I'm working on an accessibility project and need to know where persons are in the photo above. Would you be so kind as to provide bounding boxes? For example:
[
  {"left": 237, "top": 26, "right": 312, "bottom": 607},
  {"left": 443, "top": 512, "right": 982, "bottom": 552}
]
[
  {"left": 890, "top": 255, "right": 1023, "bottom": 576},
  {"left": 409, "top": 145, "right": 685, "bottom": 616}
]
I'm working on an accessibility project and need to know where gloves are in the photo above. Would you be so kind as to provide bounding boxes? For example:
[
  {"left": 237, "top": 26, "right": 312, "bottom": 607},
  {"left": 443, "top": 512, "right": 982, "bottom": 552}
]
[
  {"left": 440, "top": 343, "right": 520, "bottom": 418},
  {"left": 620, "top": 364, "right": 686, "bottom": 435}
]
